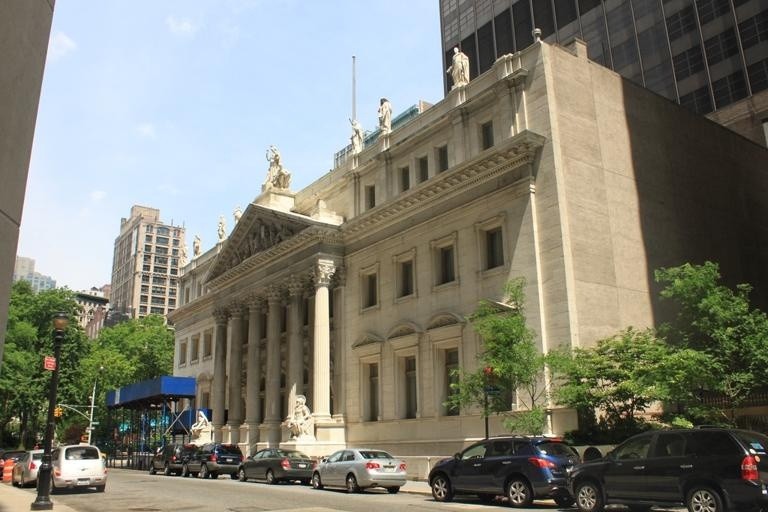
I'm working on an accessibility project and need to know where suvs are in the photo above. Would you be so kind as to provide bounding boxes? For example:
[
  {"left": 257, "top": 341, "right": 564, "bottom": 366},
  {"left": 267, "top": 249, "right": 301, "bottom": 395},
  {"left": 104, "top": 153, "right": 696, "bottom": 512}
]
[
  {"left": 568, "top": 427, "right": 768, "bottom": 512},
  {"left": 429, "top": 436, "right": 581, "bottom": 508}
]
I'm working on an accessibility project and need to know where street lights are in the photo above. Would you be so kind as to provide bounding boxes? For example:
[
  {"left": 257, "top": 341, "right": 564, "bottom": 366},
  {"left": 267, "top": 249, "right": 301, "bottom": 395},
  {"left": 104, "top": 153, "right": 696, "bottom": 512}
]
[{"left": 29, "top": 310, "right": 71, "bottom": 510}]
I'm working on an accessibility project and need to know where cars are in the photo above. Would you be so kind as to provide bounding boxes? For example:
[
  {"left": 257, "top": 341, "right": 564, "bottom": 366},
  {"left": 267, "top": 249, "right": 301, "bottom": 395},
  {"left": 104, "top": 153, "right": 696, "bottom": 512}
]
[
  {"left": 150, "top": 444, "right": 408, "bottom": 495},
  {"left": 1, "top": 445, "right": 107, "bottom": 493}
]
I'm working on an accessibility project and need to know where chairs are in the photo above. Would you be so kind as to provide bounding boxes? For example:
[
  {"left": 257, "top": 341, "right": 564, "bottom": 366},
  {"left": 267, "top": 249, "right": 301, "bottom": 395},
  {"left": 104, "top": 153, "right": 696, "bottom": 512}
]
[{"left": 487, "top": 441, "right": 505, "bottom": 457}]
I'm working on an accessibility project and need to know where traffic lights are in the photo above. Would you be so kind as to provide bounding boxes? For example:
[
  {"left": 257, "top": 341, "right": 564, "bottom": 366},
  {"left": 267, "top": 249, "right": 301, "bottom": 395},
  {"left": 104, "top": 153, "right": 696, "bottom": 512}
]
[
  {"left": 54, "top": 408, "right": 58, "bottom": 417},
  {"left": 59, "top": 407, "right": 63, "bottom": 417},
  {"left": 81, "top": 435, "right": 89, "bottom": 442}
]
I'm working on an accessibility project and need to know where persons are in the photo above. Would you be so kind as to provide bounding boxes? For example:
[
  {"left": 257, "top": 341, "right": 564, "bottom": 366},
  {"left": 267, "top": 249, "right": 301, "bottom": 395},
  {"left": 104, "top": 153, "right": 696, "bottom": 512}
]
[
  {"left": 177, "top": 248, "right": 188, "bottom": 266},
  {"left": 264, "top": 143, "right": 282, "bottom": 185},
  {"left": 444, "top": 47, "right": 471, "bottom": 92},
  {"left": 347, "top": 117, "right": 364, "bottom": 156},
  {"left": 286, "top": 394, "right": 310, "bottom": 437},
  {"left": 217, "top": 215, "right": 226, "bottom": 239},
  {"left": 231, "top": 205, "right": 243, "bottom": 225},
  {"left": 377, "top": 98, "right": 393, "bottom": 134},
  {"left": 192, "top": 234, "right": 202, "bottom": 256},
  {"left": 188, "top": 410, "right": 210, "bottom": 435}
]
